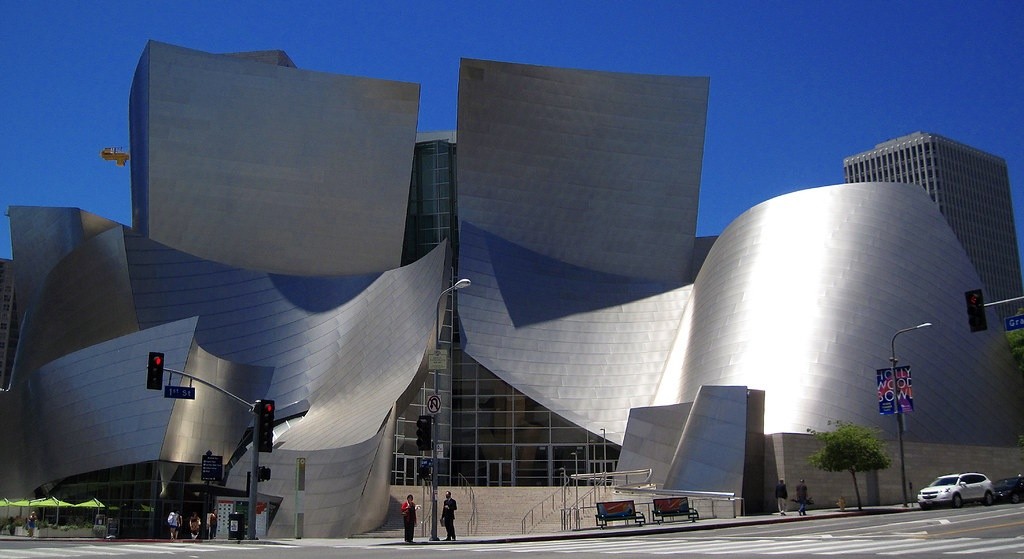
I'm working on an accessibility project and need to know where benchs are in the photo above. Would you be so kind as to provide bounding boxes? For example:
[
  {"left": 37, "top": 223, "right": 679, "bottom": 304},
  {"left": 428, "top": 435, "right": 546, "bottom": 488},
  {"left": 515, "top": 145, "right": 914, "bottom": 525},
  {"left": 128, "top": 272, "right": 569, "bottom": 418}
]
[
  {"left": 653, "top": 497, "right": 699, "bottom": 526},
  {"left": 595, "top": 500, "right": 646, "bottom": 529}
]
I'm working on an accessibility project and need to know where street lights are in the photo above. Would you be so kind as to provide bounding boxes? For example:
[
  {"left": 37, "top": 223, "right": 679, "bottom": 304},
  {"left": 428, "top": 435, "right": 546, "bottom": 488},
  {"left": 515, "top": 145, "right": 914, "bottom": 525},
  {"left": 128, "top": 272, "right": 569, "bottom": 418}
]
[
  {"left": 600, "top": 427, "right": 607, "bottom": 493},
  {"left": 427, "top": 278, "right": 473, "bottom": 544},
  {"left": 559, "top": 467, "right": 567, "bottom": 508},
  {"left": 589, "top": 438, "right": 597, "bottom": 506},
  {"left": 571, "top": 452, "right": 580, "bottom": 510},
  {"left": 889, "top": 321, "right": 934, "bottom": 507}
]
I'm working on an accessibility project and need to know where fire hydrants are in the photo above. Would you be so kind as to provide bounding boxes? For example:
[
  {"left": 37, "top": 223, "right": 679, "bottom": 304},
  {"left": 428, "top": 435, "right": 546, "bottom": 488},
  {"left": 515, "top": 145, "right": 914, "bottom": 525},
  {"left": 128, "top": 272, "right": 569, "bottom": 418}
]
[{"left": 835, "top": 496, "right": 846, "bottom": 512}]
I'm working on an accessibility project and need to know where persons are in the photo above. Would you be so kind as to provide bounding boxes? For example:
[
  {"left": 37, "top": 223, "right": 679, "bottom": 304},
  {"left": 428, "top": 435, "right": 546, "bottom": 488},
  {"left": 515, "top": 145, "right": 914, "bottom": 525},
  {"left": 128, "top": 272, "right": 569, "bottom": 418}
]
[
  {"left": 190, "top": 512, "right": 201, "bottom": 539},
  {"left": 28, "top": 511, "right": 37, "bottom": 538},
  {"left": 775, "top": 480, "right": 788, "bottom": 516},
  {"left": 796, "top": 479, "right": 808, "bottom": 516},
  {"left": 441, "top": 491, "right": 457, "bottom": 541},
  {"left": 209, "top": 509, "right": 218, "bottom": 539},
  {"left": 170, "top": 511, "right": 182, "bottom": 540},
  {"left": 402, "top": 495, "right": 416, "bottom": 543}
]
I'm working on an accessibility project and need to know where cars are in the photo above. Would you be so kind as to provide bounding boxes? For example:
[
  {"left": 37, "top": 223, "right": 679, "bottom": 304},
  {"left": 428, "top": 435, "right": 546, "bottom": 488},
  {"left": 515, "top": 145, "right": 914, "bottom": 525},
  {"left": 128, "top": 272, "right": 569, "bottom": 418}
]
[{"left": 992, "top": 476, "right": 1024, "bottom": 504}]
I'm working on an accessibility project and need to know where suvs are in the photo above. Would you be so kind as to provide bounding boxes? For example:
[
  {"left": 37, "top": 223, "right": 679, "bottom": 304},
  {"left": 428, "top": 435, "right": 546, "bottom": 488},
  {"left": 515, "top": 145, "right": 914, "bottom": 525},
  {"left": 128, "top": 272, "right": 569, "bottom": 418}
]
[{"left": 916, "top": 471, "right": 997, "bottom": 508}]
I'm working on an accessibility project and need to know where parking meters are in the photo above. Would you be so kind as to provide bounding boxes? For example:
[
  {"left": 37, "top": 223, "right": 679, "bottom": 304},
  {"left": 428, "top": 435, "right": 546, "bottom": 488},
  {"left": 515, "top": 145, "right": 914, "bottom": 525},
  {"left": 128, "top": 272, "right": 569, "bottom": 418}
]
[{"left": 909, "top": 482, "right": 914, "bottom": 507}]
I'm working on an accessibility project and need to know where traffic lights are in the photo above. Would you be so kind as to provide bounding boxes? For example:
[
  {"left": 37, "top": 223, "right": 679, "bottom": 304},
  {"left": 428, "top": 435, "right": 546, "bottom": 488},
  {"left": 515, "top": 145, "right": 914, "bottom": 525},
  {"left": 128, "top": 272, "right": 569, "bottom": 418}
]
[
  {"left": 416, "top": 416, "right": 433, "bottom": 451},
  {"left": 258, "top": 399, "right": 277, "bottom": 454},
  {"left": 259, "top": 467, "right": 270, "bottom": 481},
  {"left": 146, "top": 352, "right": 165, "bottom": 391},
  {"left": 964, "top": 288, "right": 988, "bottom": 332}
]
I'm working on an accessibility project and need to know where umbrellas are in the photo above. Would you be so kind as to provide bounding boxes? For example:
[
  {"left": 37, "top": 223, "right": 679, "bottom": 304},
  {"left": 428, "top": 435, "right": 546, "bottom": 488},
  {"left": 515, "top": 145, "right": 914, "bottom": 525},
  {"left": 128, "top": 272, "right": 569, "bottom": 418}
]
[{"left": 0, "top": 496, "right": 105, "bottom": 524}]
